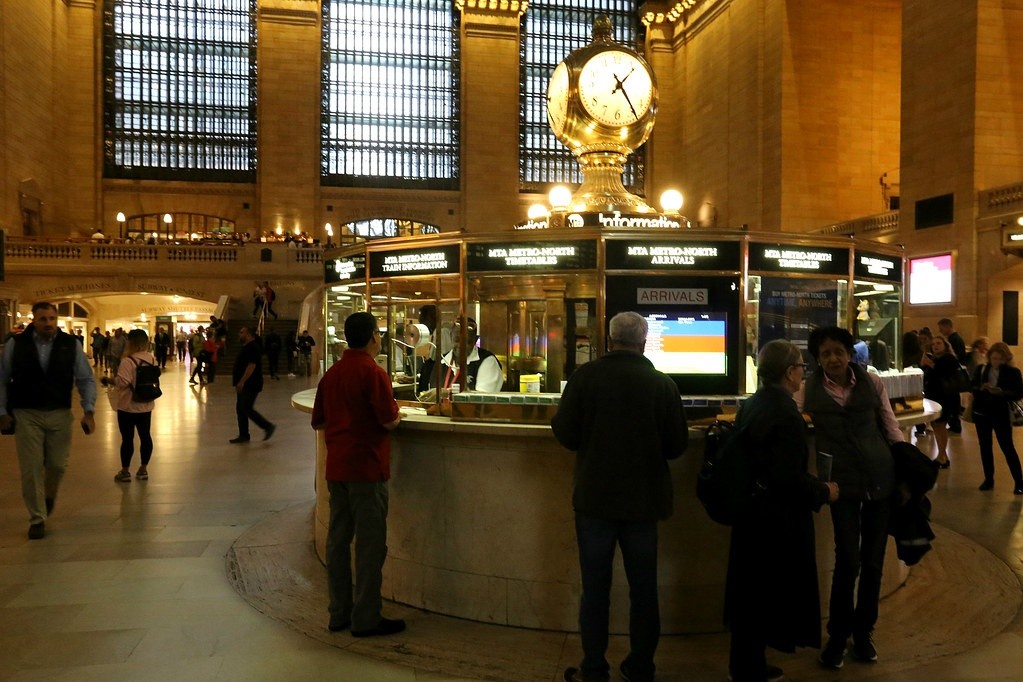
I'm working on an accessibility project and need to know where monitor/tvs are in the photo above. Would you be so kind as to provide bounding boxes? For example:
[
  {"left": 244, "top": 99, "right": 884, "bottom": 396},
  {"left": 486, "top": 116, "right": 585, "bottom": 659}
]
[{"left": 634, "top": 308, "right": 730, "bottom": 379}]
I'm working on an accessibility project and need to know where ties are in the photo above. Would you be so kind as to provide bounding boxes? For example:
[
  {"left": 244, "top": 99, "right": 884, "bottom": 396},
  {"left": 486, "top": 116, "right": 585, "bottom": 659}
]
[{"left": 784, "top": 361, "right": 809, "bottom": 371}]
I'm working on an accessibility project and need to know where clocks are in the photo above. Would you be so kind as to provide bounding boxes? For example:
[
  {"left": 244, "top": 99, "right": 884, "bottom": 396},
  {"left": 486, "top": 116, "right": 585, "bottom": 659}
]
[
  {"left": 545, "top": 60, "right": 569, "bottom": 135},
  {"left": 579, "top": 49, "right": 652, "bottom": 127}
]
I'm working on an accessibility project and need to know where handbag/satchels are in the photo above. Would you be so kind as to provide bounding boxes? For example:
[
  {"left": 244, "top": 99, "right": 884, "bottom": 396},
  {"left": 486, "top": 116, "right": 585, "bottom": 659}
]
[
  {"left": 938, "top": 356, "right": 972, "bottom": 391},
  {"left": 0, "top": 334, "right": 22, "bottom": 435},
  {"left": 1004, "top": 388, "right": 1023, "bottom": 426}
]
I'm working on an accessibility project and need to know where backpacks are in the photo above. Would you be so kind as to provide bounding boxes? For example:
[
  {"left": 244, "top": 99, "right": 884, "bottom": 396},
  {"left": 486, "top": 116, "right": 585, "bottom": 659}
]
[
  {"left": 127, "top": 356, "right": 162, "bottom": 403},
  {"left": 695, "top": 398, "right": 804, "bottom": 528}
]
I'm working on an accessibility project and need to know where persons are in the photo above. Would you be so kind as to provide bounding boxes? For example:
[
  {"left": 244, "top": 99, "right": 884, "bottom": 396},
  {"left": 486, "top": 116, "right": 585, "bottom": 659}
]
[
  {"left": 311, "top": 312, "right": 404, "bottom": 637},
  {"left": 229, "top": 325, "right": 276, "bottom": 442},
  {"left": 792, "top": 327, "right": 907, "bottom": 668},
  {"left": 0, "top": 302, "right": 97, "bottom": 540},
  {"left": 99, "top": 329, "right": 158, "bottom": 480},
  {"left": 723, "top": 339, "right": 839, "bottom": 682},
  {"left": 284, "top": 327, "right": 299, "bottom": 376},
  {"left": 869, "top": 311, "right": 1023, "bottom": 494},
  {"left": 297, "top": 331, "right": 315, "bottom": 376},
  {"left": 552, "top": 311, "right": 690, "bottom": 682},
  {"left": 432, "top": 317, "right": 505, "bottom": 392},
  {"left": 5, "top": 280, "right": 278, "bottom": 384},
  {"left": 92, "top": 229, "right": 336, "bottom": 250},
  {"left": 418, "top": 305, "right": 450, "bottom": 393}
]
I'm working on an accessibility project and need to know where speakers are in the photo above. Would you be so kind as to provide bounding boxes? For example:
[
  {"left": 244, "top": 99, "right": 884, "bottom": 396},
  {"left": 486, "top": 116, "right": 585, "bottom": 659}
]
[{"left": 261, "top": 248, "right": 272, "bottom": 262}]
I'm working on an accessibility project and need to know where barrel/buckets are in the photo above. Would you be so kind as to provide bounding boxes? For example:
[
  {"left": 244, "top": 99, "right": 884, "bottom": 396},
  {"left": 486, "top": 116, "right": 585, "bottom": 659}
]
[{"left": 520, "top": 374, "right": 540, "bottom": 394}]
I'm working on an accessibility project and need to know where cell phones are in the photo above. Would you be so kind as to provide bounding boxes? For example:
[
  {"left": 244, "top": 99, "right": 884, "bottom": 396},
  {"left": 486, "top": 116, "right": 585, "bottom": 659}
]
[
  {"left": 926, "top": 352, "right": 932, "bottom": 358},
  {"left": 82, "top": 423, "right": 88, "bottom": 433}
]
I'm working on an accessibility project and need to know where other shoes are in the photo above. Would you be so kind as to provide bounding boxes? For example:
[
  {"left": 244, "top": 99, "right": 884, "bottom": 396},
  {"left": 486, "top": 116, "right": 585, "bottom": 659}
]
[
  {"left": 116, "top": 471, "right": 131, "bottom": 482},
  {"left": 263, "top": 425, "right": 274, "bottom": 441},
  {"left": 230, "top": 436, "right": 250, "bottom": 442},
  {"left": 979, "top": 479, "right": 993, "bottom": 491},
  {"left": 136, "top": 470, "right": 148, "bottom": 479},
  {"left": 28, "top": 520, "right": 44, "bottom": 539},
  {"left": 818, "top": 637, "right": 849, "bottom": 669},
  {"left": 563, "top": 667, "right": 587, "bottom": 682},
  {"left": 619, "top": 661, "right": 634, "bottom": 682},
  {"left": 1014, "top": 480, "right": 1023, "bottom": 495},
  {"left": 850, "top": 625, "right": 878, "bottom": 662},
  {"left": 728, "top": 664, "right": 783, "bottom": 682},
  {"left": 933, "top": 458, "right": 950, "bottom": 469},
  {"left": 350, "top": 606, "right": 405, "bottom": 637},
  {"left": 329, "top": 603, "right": 355, "bottom": 632},
  {"left": 46, "top": 496, "right": 54, "bottom": 516}
]
